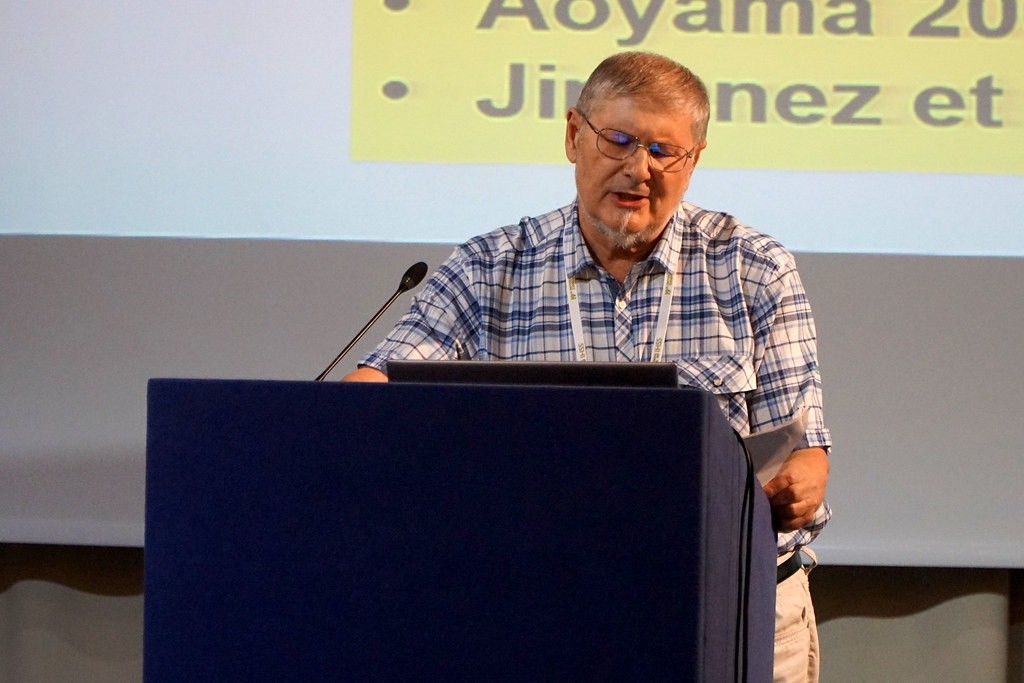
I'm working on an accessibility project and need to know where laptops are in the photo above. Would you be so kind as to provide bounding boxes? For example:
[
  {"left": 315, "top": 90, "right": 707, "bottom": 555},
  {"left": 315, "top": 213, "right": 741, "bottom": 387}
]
[{"left": 384, "top": 359, "right": 679, "bottom": 390}]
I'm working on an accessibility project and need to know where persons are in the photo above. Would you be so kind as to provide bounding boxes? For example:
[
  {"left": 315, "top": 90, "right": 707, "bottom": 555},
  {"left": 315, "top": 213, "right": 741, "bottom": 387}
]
[{"left": 339, "top": 50, "right": 832, "bottom": 683}]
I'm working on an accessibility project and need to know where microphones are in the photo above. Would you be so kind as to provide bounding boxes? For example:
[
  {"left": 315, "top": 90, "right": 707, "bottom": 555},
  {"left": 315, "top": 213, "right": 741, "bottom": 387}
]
[{"left": 312, "top": 262, "right": 428, "bottom": 382}]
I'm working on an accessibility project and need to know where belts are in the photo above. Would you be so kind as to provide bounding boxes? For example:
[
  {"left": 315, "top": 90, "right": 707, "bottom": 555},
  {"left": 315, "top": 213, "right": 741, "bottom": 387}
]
[{"left": 776, "top": 551, "right": 802, "bottom": 584}]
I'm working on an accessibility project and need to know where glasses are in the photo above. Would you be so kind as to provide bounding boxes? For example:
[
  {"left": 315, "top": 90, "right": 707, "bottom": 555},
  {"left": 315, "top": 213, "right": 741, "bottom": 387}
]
[{"left": 581, "top": 111, "right": 698, "bottom": 172}]
